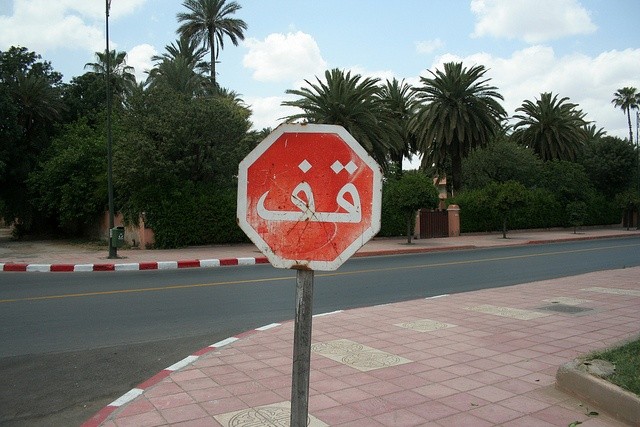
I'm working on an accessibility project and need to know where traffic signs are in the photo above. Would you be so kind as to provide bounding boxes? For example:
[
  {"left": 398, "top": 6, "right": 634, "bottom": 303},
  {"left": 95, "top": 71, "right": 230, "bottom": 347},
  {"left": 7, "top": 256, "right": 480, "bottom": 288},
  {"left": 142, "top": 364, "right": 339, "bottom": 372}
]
[{"left": 236, "top": 122, "right": 382, "bottom": 271}]
